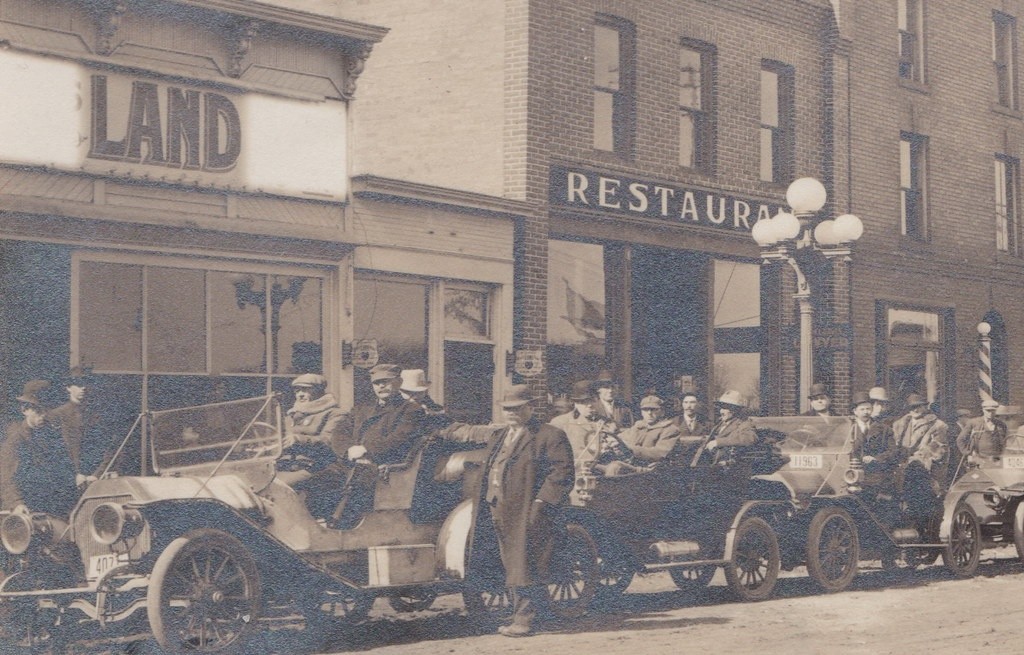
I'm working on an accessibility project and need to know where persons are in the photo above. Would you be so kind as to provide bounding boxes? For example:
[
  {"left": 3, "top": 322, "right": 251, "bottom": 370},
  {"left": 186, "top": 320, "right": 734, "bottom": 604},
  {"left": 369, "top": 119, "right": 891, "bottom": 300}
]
[
  {"left": 468, "top": 386, "right": 575, "bottom": 638},
  {"left": 248, "top": 373, "right": 353, "bottom": 473},
  {"left": 301, "top": 364, "right": 427, "bottom": 528},
  {"left": 619, "top": 396, "right": 682, "bottom": 471},
  {"left": 704, "top": 391, "right": 758, "bottom": 462},
  {"left": 800, "top": 384, "right": 836, "bottom": 416},
  {"left": 400, "top": 368, "right": 446, "bottom": 416},
  {"left": 181, "top": 383, "right": 252, "bottom": 465},
  {"left": 893, "top": 394, "right": 1006, "bottom": 523},
  {"left": 0, "top": 366, "right": 127, "bottom": 655},
  {"left": 548, "top": 381, "right": 634, "bottom": 507},
  {"left": 671, "top": 390, "right": 707, "bottom": 437},
  {"left": 850, "top": 388, "right": 896, "bottom": 517},
  {"left": 596, "top": 369, "right": 631, "bottom": 427}
]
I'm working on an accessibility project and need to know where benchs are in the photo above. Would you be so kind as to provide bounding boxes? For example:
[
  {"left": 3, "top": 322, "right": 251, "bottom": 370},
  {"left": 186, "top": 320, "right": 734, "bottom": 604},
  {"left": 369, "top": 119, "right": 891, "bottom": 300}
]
[
  {"left": 356, "top": 434, "right": 434, "bottom": 510},
  {"left": 638, "top": 432, "right": 776, "bottom": 500},
  {"left": 429, "top": 438, "right": 490, "bottom": 499}
]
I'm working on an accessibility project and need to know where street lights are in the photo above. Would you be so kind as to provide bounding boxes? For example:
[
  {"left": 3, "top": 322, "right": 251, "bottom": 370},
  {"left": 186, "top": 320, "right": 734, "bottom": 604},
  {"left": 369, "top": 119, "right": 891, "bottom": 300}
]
[{"left": 751, "top": 176, "right": 865, "bottom": 418}]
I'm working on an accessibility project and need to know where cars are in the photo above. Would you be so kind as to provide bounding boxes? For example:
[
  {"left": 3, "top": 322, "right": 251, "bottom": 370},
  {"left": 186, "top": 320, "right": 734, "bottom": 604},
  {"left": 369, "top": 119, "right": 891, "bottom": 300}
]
[
  {"left": 939, "top": 433, "right": 1024, "bottom": 560},
  {"left": 746, "top": 415, "right": 953, "bottom": 594},
  {"left": 0, "top": 414, "right": 535, "bottom": 655},
  {"left": 536, "top": 427, "right": 799, "bottom": 621}
]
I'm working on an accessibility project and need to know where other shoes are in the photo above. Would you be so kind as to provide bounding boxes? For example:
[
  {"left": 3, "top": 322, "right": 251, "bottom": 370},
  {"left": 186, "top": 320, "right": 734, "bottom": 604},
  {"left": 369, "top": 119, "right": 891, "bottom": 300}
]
[{"left": 498, "top": 622, "right": 534, "bottom": 636}]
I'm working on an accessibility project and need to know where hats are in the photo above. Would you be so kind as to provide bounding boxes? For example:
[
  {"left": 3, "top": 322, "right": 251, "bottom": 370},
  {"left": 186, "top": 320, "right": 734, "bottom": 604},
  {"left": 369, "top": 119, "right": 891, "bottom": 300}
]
[
  {"left": 713, "top": 390, "right": 746, "bottom": 411},
  {"left": 568, "top": 381, "right": 599, "bottom": 401},
  {"left": 678, "top": 386, "right": 699, "bottom": 400},
  {"left": 807, "top": 383, "right": 832, "bottom": 400},
  {"left": 954, "top": 409, "right": 970, "bottom": 417},
  {"left": 849, "top": 391, "right": 871, "bottom": 412},
  {"left": 495, "top": 385, "right": 542, "bottom": 406},
  {"left": 592, "top": 369, "right": 616, "bottom": 382},
  {"left": 398, "top": 368, "right": 433, "bottom": 391},
  {"left": 905, "top": 394, "right": 928, "bottom": 407},
  {"left": 638, "top": 396, "right": 664, "bottom": 411},
  {"left": 15, "top": 379, "right": 55, "bottom": 407},
  {"left": 869, "top": 387, "right": 888, "bottom": 402},
  {"left": 58, "top": 365, "right": 92, "bottom": 385},
  {"left": 368, "top": 365, "right": 400, "bottom": 382},
  {"left": 291, "top": 373, "right": 328, "bottom": 391},
  {"left": 980, "top": 400, "right": 1000, "bottom": 409}
]
